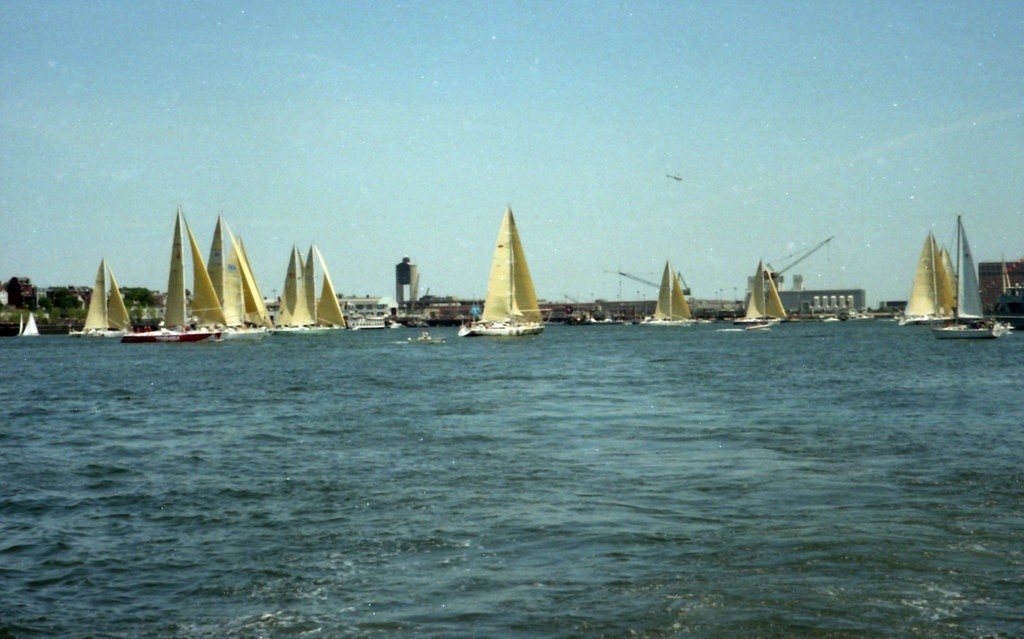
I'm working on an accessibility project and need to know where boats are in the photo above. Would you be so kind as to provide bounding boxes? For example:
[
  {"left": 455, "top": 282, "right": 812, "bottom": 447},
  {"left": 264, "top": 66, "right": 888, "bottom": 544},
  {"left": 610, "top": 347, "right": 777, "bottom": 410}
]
[{"left": 348, "top": 315, "right": 385, "bottom": 330}]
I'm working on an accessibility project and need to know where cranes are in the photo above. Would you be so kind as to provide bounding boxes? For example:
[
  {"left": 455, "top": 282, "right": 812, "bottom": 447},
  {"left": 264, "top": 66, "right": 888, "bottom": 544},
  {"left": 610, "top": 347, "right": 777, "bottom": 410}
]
[
  {"left": 768, "top": 235, "right": 836, "bottom": 278},
  {"left": 616, "top": 269, "right": 692, "bottom": 296}
]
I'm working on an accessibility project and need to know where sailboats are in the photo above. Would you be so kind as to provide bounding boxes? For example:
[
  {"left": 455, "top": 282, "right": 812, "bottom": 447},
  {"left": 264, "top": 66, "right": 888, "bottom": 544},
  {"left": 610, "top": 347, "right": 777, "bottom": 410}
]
[
  {"left": 266, "top": 244, "right": 349, "bottom": 336},
  {"left": 458, "top": 204, "right": 545, "bottom": 337},
  {"left": 120, "top": 205, "right": 273, "bottom": 345},
  {"left": 733, "top": 260, "right": 789, "bottom": 323},
  {"left": 896, "top": 212, "right": 1024, "bottom": 339},
  {"left": 66, "top": 258, "right": 132, "bottom": 338},
  {"left": 637, "top": 260, "right": 697, "bottom": 327},
  {"left": 14, "top": 312, "right": 42, "bottom": 337}
]
